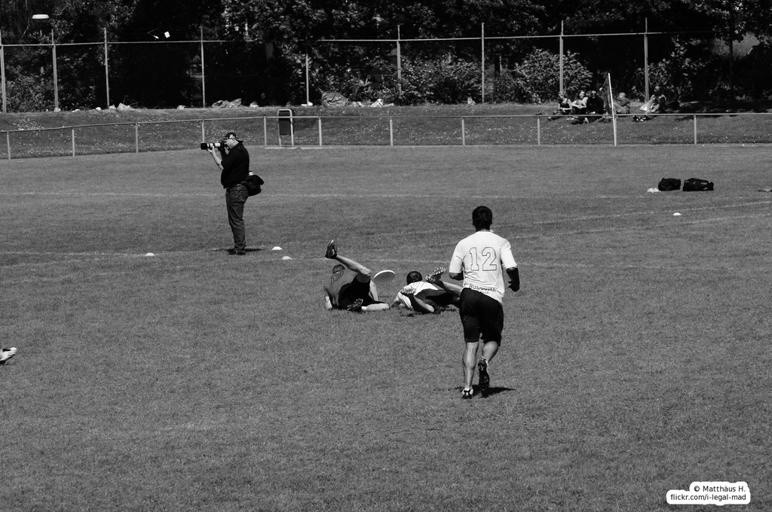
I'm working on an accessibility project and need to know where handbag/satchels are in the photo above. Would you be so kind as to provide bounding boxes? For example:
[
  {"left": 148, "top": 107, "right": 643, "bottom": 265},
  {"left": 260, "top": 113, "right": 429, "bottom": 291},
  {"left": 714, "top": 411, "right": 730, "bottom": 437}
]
[
  {"left": 244, "top": 175, "right": 264, "bottom": 196},
  {"left": 658, "top": 177, "right": 680, "bottom": 190},
  {"left": 683, "top": 177, "right": 713, "bottom": 191}
]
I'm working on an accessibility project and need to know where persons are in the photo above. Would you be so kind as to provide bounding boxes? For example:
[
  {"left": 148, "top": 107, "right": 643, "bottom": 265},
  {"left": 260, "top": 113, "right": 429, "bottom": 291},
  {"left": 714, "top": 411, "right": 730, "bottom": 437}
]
[
  {"left": 548, "top": 84, "right": 667, "bottom": 126},
  {"left": 207, "top": 132, "right": 249, "bottom": 255},
  {"left": 449, "top": 206, "right": 521, "bottom": 397},
  {"left": 323, "top": 238, "right": 390, "bottom": 312},
  {"left": 389, "top": 266, "right": 463, "bottom": 315}
]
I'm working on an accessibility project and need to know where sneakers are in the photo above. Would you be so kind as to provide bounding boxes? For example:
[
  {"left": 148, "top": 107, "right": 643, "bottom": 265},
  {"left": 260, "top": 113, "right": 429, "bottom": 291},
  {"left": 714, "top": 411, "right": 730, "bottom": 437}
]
[
  {"left": 478, "top": 356, "right": 489, "bottom": 398},
  {"left": 324, "top": 239, "right": 337, "bottom": 259},
  {"left": 424, "top": 266, "right": 447, "bottom": 283},
  {"left": 227, "top": 248, "right": 245, "bottom": 255},
  {"left": 461, "top": 386, "right": 474, "bottom": 399},
  {"left": 400, "top": 287, "right": 416, "bottom": 295}
]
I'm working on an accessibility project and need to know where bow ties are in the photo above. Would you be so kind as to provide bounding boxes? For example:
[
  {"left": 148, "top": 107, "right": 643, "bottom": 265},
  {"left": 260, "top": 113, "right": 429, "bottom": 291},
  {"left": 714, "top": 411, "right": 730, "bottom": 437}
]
[{"left": 346, "top": 298, "right": 364, "bottom": 312}]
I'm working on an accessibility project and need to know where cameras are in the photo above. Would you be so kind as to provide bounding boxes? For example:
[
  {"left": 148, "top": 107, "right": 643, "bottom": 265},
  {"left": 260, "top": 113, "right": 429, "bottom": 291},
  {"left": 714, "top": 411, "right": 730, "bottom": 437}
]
[{"left": 201, "top": 141, "right": 225, "bottom": 150}]
